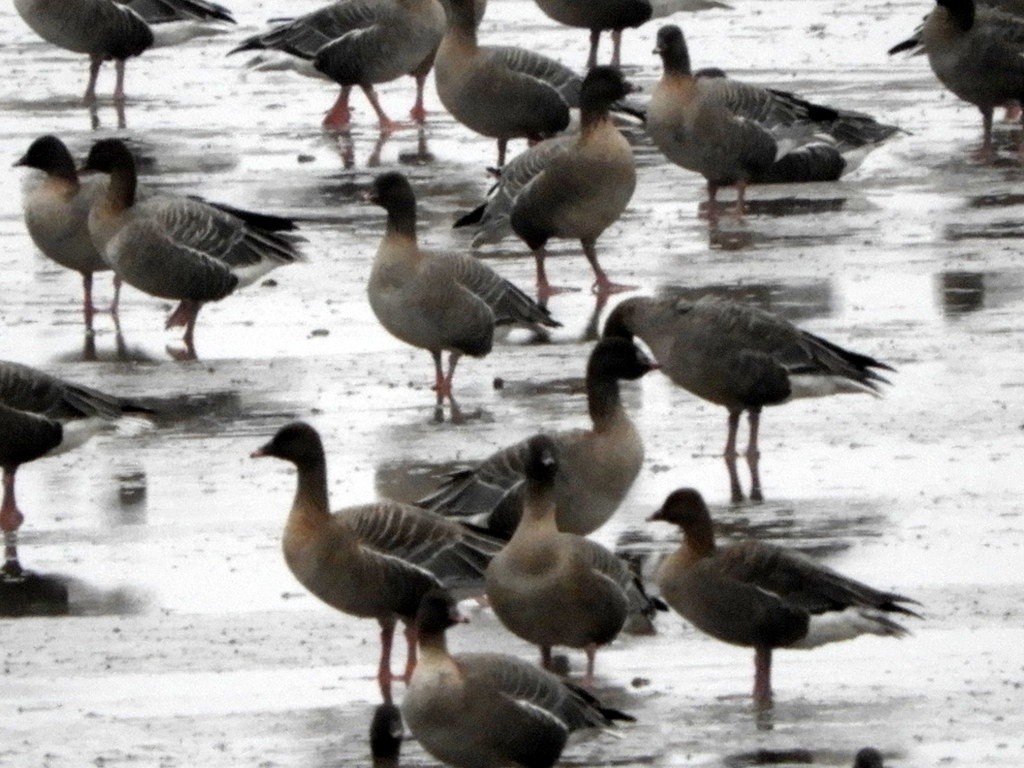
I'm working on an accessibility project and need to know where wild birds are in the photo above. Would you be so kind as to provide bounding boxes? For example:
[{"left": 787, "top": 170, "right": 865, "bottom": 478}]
[
  {"left": 451, "top": 66, "right": 642, "bottom": 313},
  {"left": 401, "top": 588, "right": 639, "bottom": 768},
  {"left": 483, "top": 434, "right": 672, "bottom": 699},
  {"left": 250, "top": 420, "right": 509, "bottom": 685},
  {"left": 358, "top": 169, "right": 568, "bottom": 403},
  {"left": 599, "top": 293, "right": 902, "bottom": 506},
  {"left": 12, "top": 0, "right": 1024, "bottom": 221},
  {"left": 0, "top": 360, "right": 167, "bottom": 542},
  {"left": 12, "top": 135, "right": 309, "bottom": 362},
  {"left": 410, "top": 337, "right": 665, "bottom": 543},
  {"left": 644, "top": 487, "right": 928, "bottom": 717}
]
